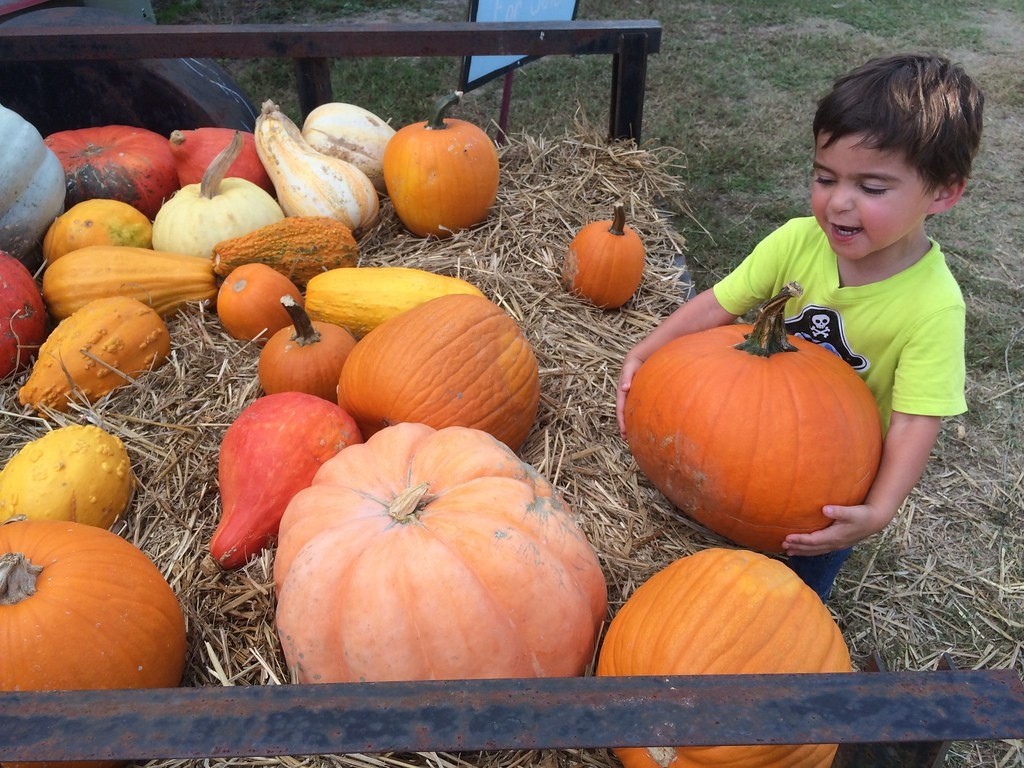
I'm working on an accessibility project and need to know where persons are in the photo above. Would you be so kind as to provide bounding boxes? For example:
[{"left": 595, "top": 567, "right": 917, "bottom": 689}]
[{"left": 616, "top": 53, "right": 986, "bottom": 604}]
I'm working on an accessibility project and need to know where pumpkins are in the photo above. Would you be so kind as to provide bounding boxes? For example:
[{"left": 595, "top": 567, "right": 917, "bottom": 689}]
[
  {"left": 621, "top": 280, "right": 883, "bottom": 552},
  {"left": 596, "top": 546, "right": 852, "bottom": 768},
  {"left": 0, "top": 90, "right": 653, "bottom": 768}
]
